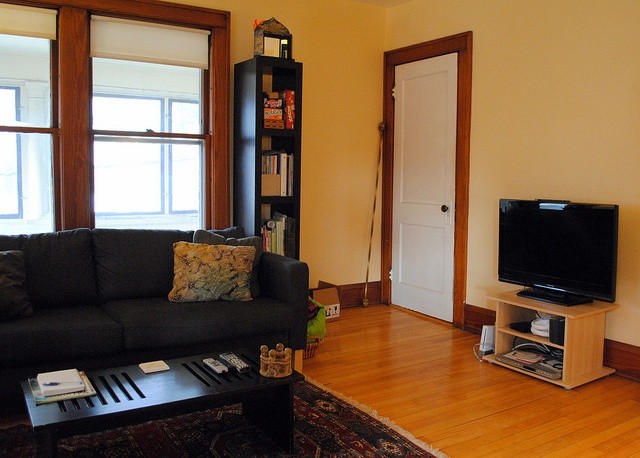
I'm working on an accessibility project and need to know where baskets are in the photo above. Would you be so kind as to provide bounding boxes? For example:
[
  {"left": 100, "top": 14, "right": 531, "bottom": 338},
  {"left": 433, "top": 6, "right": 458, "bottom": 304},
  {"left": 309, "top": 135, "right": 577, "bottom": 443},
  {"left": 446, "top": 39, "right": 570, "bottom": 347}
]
[{"left": 302, "top": 334, "right": 325, "bottom": 361}]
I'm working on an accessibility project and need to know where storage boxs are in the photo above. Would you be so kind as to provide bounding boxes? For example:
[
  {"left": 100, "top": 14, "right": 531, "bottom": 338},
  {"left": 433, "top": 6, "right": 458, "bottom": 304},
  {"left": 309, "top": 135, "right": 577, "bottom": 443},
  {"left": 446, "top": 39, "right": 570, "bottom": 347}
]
[{"left": 310, "top": 280, "right": 343, "bottom": 323}]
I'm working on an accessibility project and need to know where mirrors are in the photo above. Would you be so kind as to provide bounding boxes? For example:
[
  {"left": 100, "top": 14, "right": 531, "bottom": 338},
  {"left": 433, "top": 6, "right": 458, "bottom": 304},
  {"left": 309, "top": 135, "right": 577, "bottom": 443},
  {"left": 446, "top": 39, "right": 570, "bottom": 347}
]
[{"left": 254, "top": 17, "right": 292, "bottom": 59}]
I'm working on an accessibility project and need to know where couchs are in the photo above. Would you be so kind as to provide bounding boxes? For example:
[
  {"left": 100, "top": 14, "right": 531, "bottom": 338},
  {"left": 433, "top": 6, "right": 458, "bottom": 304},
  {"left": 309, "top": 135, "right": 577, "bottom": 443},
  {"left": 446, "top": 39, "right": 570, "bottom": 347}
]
[{"left": 0, "top": 227, "right": 309, "bottom": 415}]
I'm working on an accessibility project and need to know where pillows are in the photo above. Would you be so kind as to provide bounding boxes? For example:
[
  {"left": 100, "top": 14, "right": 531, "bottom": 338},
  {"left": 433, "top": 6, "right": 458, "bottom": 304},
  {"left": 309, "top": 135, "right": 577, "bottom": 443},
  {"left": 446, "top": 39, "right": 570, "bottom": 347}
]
[
  {"left": 0, "top": 250, "right": 35, "bottom": 318},
  {"left": 193, "top": 229, "right": 263, "bottom": 298},
  {"left": 167, "top": 240, "right": 256, "bottom": 302}
]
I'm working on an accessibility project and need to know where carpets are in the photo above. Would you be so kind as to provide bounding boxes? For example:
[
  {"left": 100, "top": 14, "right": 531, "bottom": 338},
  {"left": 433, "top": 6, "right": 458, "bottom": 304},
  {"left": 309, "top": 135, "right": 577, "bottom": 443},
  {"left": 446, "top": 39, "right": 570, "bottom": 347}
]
[{"left": 0, "top": 377, "right": 448, "bottom": 457}]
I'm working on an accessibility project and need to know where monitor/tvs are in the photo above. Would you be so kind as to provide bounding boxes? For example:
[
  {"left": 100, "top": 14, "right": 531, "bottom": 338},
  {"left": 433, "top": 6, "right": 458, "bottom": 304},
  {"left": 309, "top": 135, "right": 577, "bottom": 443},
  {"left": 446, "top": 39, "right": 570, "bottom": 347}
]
[{"left": 498, "top": 196, "right": 620, "bottom": 308}]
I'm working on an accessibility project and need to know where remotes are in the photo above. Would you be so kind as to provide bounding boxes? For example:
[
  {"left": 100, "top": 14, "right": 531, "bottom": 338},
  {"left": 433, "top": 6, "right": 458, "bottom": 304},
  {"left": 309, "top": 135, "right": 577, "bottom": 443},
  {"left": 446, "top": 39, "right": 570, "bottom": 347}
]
[
  {"left": 204, "top": 358, "right": 228, "bottom": 374},
  {"left": 220, "top": 351, "right": 252, "bottom": 374},
  {"left": 215, "top": 356, "right": 236, "bottom": 374}
]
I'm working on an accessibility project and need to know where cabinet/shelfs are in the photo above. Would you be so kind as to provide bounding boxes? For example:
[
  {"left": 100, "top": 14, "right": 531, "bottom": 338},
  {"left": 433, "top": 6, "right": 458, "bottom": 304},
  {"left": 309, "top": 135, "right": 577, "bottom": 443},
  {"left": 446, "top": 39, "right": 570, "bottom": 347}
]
[
  {"left": 233, "top": 56, "right": 303, "bottom": 261},
  {"left": 482, "top": 288, "right": 620, "bottom": 390}
]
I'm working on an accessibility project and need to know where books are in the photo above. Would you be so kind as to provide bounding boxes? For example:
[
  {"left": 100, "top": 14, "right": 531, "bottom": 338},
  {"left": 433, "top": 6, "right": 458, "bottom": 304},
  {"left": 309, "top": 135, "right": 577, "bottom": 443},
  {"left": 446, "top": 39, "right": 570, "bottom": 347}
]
[
  {"left": 261, "top": 151, "right": 293, "bottom": 197},
  {"left": 263, "top": 212, "right": 295, "bottom": 257},
  {"left": 28, "top": 368, "right": 98, "bottom": 404}
]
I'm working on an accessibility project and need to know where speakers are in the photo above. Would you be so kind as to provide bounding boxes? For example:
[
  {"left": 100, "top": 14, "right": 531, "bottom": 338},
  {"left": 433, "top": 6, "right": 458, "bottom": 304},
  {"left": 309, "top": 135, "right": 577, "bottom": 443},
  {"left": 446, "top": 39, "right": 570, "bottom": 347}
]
[{"left": 549, "top": 317, "right": 565, "bottom": 346}]
